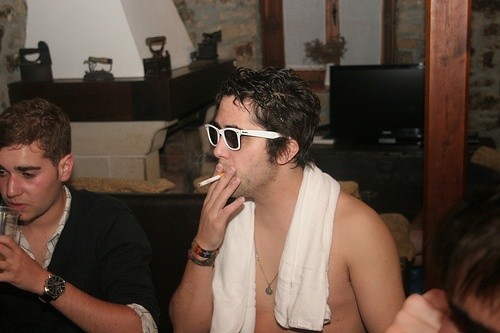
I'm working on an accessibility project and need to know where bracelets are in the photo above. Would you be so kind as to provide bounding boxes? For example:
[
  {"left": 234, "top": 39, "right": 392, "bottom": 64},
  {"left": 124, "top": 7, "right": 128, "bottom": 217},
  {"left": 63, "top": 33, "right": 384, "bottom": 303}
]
[{"left": 188, "top": 239, "right": 217, "bottom": 266}]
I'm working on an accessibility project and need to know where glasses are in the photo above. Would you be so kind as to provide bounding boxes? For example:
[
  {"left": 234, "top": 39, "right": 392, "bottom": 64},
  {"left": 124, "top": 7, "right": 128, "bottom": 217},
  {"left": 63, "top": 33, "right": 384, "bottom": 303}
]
[{"left": 205, "top": 124, "right": 282, "bottom": 150}]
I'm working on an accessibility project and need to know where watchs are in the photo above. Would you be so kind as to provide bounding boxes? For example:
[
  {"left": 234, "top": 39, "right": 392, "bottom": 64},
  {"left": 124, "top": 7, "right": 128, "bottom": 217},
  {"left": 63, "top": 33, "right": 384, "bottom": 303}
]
[{"left": 38, "top": 274, "right": 66, "bottom": 304}]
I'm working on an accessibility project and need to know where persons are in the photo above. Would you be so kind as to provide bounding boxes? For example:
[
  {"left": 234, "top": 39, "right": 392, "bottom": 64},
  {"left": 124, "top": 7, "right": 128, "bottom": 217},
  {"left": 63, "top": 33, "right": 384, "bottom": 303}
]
[
  {"left": 0, "top": 97, "right": 160, "bottom": 333},
  {"left": 385, "top": 184, "right": 500, "bottom": 333},
  {"left": 169, "top": 66, "right": 406, "bottom": 333}
]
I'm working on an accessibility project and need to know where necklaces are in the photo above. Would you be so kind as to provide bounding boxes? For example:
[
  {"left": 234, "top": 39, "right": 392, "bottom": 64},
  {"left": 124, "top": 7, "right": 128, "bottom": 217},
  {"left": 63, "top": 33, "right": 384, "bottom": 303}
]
[{"left": 256, "top": 247, "right": 278, "bottom": 295}]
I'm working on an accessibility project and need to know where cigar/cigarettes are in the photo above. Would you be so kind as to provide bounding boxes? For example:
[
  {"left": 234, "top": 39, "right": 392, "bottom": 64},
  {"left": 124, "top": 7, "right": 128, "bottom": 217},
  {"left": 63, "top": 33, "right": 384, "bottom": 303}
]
[{"left": 198, "top": 173, "right": 225, "bottom": 188}]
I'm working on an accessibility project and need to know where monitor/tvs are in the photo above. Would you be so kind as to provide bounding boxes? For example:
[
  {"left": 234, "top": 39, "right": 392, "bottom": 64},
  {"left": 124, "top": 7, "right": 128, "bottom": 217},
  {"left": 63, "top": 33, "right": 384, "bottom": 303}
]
[{"left": 330, "top": 64, "right": 426, "bottom": 148}]
